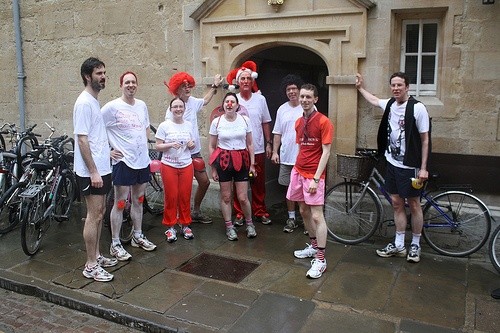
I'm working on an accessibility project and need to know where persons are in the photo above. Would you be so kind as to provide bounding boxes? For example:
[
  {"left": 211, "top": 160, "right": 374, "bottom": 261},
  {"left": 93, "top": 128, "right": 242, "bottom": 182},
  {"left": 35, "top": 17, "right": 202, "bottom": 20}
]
[
  {"left": 226, "top": 61, "right": 273, "bottom": 227},
  {"left": 286, "top": 83, "right": 334, "bottom": 278},
  {"left": 271, "top": 75, "right": 317, "bottom": 236},
  {"left": 208, "top": 92, "right": 257, "bottom": 241},
  {"left": 100, "top": 71, "right": 158, "bottom": 261},
  {"left": 154, "top": 97, "right": 197, "bottom": 243},
  {"left": 163, "top": 73, "right": 224, "bottom": 227},
  {"left": 355, "top": 72, "right": 432, "bottom": 262},
  {"left": 73, "top": 57, "right": 119, "bottom": 282}
]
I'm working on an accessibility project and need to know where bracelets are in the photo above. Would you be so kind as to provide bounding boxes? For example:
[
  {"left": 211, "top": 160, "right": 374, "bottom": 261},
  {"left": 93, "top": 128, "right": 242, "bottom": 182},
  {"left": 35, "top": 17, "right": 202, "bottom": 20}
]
[{"left": 250, "top": 163, "right": 258, "bottom": 168}]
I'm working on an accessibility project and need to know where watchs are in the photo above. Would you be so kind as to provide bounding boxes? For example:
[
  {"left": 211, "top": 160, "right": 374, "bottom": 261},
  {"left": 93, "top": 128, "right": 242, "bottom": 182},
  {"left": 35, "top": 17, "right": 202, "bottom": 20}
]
[
  {"left": 211, "top": 83, "right": 218, "bottom": 89},
  {"left": 313, "top": 178, "right": 320, "bottom": 183},
  {"left": 266, "top": 140, "right": 272, "bottom": 145}
]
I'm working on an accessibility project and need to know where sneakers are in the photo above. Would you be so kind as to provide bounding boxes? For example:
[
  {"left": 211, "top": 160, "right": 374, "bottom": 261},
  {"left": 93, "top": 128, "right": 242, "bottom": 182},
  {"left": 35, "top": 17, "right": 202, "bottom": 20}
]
[
  {"left": 294, "top": 245, "right": 317, "bottom": 258},
  {"left": 190, "top": 210, "right": 212, "bottom": 224},
  {"left": 109, "top": 242, "right": 132, "bottom": 261},
  {"left": 407, "top": 244, "right": 421, "bottom": 262},
  {"left": 305, "top": 258, "right": 327, "bottom": 279},
  {"left": 376, "top": 242, "right": 407, "bottom": 257},
  {"left": 233, "top": 215, "right": 244, "bottom": 225},
  {"left": 180, "top": 225, "right": 195, "bottom": 240},
  {"left": 225, "top": 226, "right": 239, "bottom": 240},
  {"left": 167, "top": 228, "right": 177, "bottom": 242},
  {"left": 245, "top": 223, "right": 256, "bottom": 238},
  {"left": 255, "top": 214, "right": 272, "bottom": 224},
  {"left": 96, "top": 255, "right": 118, "bottom": 267},
  {"left": 131, "top": 234, "right": 157, "bottom": 252},
  {"left": 82, "top": 261, "right": 114, "bottom": 282},
  {"left": 282, "top": 217, "right": 299, "bottom": 233}
]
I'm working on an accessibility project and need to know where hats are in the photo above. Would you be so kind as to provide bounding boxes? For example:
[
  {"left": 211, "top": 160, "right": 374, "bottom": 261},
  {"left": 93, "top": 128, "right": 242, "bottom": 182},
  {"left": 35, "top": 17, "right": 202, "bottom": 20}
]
[{"left": 226, "top": 60, "right": 262, "bottom": 95}]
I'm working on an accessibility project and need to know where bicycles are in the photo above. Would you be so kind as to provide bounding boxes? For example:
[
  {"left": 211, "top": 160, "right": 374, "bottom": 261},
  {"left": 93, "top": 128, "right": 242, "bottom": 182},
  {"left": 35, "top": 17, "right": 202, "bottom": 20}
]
[
  {"left": 1, "top": 119, "right": 80, "bottom": 259},
  {"left": 321, "top": 137, "right": 496, "bottom": 257},
  {"left": 102, "top": 122, "right": 168, "bottom": 242}
]
